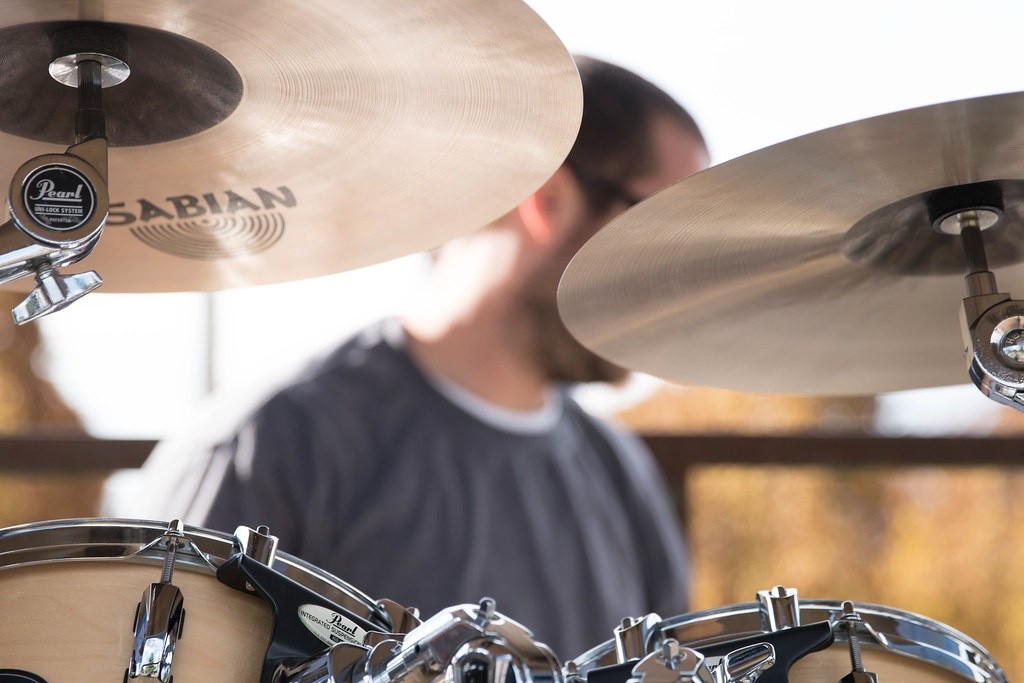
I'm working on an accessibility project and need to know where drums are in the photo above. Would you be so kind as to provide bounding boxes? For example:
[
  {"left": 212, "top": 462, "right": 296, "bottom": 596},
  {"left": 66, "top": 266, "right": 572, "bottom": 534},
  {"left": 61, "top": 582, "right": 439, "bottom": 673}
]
[
  {"left": 0, "top": 519, "right": 448, "bottom": 682},
  {"left": 561, "top": 583, "right": 1013, "bottom": 682}
]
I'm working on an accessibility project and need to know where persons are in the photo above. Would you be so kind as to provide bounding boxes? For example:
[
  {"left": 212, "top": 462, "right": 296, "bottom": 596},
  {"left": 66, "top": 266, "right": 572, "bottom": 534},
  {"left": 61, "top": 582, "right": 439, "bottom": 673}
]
[{"left": 138, "top": 54, "right": 712, "bottom": 683}]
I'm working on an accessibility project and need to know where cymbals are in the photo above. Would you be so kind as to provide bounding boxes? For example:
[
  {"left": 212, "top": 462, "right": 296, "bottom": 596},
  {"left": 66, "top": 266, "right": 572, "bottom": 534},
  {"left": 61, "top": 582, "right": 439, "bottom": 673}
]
[
  {"left": 0, "top": 1, "right": 586, "bottom": 296},
  {"left": 554, "top": 90, "right": 1023, "bottom": 397}
]
